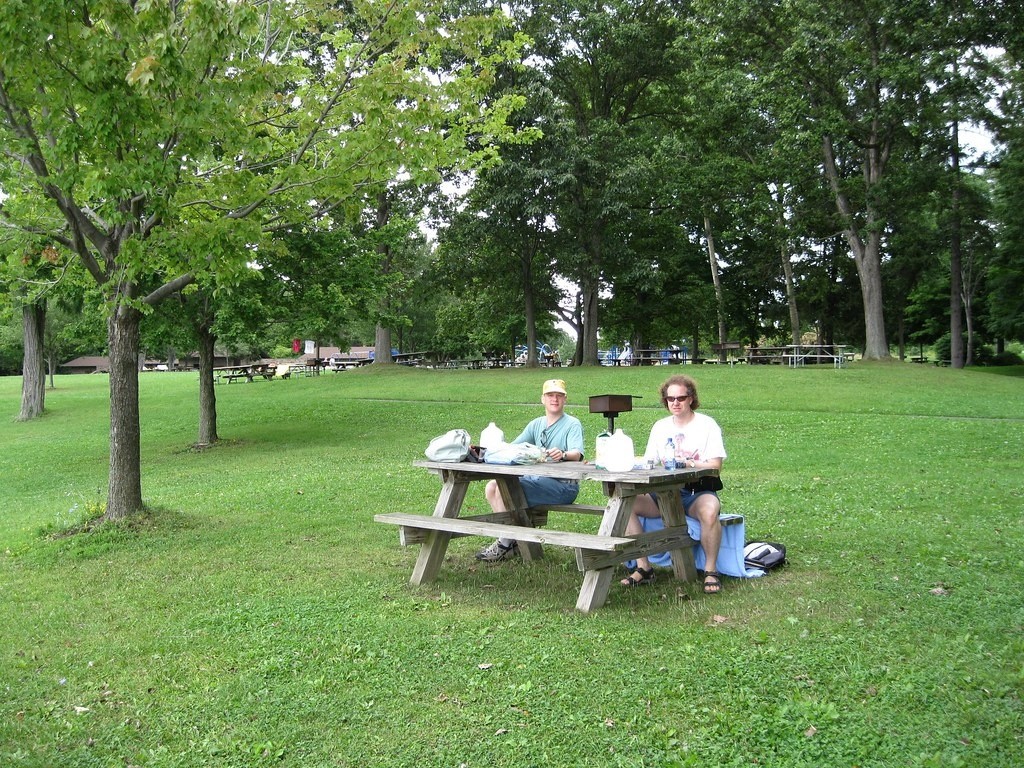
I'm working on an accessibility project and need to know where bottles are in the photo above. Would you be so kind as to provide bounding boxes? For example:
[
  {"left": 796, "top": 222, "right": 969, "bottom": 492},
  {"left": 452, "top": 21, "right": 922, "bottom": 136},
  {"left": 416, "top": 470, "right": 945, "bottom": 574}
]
[
  {"left": 664, "top": 438, "right": 676, "bottom": 471},
  {"left": 604, "top": 428, "right": 637, "bottom": 473},
  {"left": 479, "top": 422, "right": 505, "bottom": 461}
]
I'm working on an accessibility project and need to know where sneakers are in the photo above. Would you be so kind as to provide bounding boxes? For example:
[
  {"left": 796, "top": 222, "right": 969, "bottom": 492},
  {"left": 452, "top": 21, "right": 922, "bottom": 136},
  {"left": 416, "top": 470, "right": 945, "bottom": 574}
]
[
  {"left": 512, "top": 541, "right": 520, "bottom": 555},
  {"left": 475, "top": 538, "right": 514, "bottom": 562}
]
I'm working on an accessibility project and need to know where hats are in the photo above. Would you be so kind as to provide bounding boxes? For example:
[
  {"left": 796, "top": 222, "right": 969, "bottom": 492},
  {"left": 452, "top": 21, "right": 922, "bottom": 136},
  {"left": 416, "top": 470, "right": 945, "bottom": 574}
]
[{"left": 543, "top": 379, "right": 566, "bottom": 395}]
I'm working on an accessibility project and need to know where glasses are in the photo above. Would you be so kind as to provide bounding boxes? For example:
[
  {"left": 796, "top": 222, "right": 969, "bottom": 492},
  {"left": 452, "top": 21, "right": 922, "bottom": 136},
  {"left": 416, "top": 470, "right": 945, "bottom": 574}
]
[{"left": 666, "top": 395, "right": 691, "bottom": 403}]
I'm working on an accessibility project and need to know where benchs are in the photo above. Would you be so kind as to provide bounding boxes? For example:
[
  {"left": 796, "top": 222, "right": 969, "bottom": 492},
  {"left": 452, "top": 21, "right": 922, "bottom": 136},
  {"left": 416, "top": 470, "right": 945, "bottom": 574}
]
[
  {"left": 782, "top": 355, "right": 844, "bottom": 368},
  {"left": 911, "top": 361, "right": 929, "bottom": 363},
  {"left": 746, "top": 356, "right": 784, "bottom": 365},
  {"left": 633, "top": 357, "right": 675, "bottom": 366},
  {"left": 547, "top": 505, "right": 744, "bottom": 527},
  {"left": 468, "top": 362, "right": 513, "bottom": 370},
  {"left": 332, "top": 369, "right": 347, "bottom": 373},
  {"left": 293, "top": 370, "right": 322, "bottom": 379},
  {"left": 489, "top": 360, "right": 502, "bottom": 366},
  {"left": 214, "top": 372, "right": 275, "bottom": 384},
  {"left": 372, "top": 512, "right": 636, "bottom": 553}
]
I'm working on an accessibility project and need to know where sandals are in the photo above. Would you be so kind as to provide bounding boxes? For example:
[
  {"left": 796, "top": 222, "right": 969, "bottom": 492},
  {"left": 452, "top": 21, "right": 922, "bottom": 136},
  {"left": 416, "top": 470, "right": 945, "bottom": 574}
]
[
  {"left": 618, "top": 567, "right": 655, "bottom": 587},
  {"left": 703, "top": 571, "right": 721, "bottom": 594}
]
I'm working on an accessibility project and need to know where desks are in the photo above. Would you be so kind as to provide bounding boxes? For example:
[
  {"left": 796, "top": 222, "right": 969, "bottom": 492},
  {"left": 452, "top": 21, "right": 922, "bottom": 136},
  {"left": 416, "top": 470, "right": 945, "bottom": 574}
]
[
  {"left": 245, "top": 364, "right": 270, "bottom": 382},
  {"left": 410, "top": 460, "right": 720, "bottom": 612},
  {"left": 636, "top": 349, "right": 683, "bottom": 366},
  {"left": 335, "top": 362, "right": 359, "bottom": 373},
  {"left": 786, "top": 345, "right": 846, "bottom": 369},
  {"left": 213, "top": 366, "right": 250, "bottom": 385},
  {"left": 289, "top": 365, "right": 311, "bottom": 379},
  {"left": 747, "top": 347, "right": 790, "bottom": 364},
  {"left": 911, "top": 357, "right": 929, "bottom": 363},
  {"left": 469, "top": 360, "right": 486, "bottom": 369}
]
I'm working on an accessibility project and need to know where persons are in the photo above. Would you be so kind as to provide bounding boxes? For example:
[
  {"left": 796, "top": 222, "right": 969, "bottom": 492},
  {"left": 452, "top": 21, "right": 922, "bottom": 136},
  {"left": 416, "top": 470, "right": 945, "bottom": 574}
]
[
  {"left": 490, "top": 348, "right": 507, "bottom": 366},
  {"left": 396, "top": 356, "right": 418, "bottom": 367},
  {"left": 620, "top": 375, "right": 728, "bottom": 594},
  {"left": 475, "top": 379, "right": 586, "bottom": 562},
  {"left": 324, "top": 357, "right": 335, "bottom": 367}
]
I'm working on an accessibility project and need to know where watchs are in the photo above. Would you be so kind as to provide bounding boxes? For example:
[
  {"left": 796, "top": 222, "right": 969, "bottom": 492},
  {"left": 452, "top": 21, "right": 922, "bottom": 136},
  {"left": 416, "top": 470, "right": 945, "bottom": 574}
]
[{"left": 560, "top": 450, "right": 567, "bottom": 462}]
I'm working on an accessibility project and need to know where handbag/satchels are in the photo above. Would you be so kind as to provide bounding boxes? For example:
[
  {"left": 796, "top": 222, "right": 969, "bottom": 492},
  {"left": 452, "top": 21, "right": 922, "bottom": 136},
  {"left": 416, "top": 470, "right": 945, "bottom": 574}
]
[
  {"left": 743, "top": 540, "right": 786, "bottom": 571},
  {"left": 484, "top": 441, "right": 542, "bottom": 466},
  {"left": 424, "top": 429, "right": 471, "bottom": 463}
]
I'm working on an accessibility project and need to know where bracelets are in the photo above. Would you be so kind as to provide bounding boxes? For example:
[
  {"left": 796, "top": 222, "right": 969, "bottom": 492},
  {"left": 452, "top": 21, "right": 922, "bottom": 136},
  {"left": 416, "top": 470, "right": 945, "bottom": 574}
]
[{"left": 689, "top": 460, "right": 695, "bottom": 467}]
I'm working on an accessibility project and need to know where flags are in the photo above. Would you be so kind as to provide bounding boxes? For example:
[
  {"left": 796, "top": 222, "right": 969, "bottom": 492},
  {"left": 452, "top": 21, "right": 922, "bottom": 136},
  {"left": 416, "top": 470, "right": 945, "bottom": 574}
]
[
  {"left": 293, "top": 338, "right": 301, "bottom": 352},
  {"left": 304, "top": 340, "right": 315, "bottom": 354}
]
